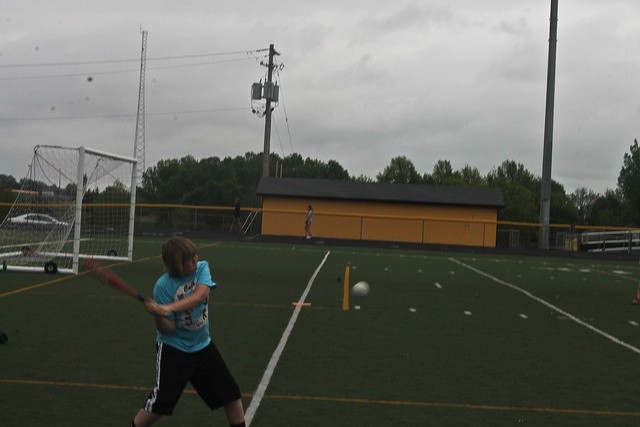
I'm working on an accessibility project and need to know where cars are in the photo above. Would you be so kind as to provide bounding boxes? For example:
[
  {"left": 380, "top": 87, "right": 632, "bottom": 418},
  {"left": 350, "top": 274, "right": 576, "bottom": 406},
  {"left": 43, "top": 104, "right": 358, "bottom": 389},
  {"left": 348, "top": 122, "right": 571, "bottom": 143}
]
[{"left": 7, "top": 213, "right": 68, "bottom": 225}]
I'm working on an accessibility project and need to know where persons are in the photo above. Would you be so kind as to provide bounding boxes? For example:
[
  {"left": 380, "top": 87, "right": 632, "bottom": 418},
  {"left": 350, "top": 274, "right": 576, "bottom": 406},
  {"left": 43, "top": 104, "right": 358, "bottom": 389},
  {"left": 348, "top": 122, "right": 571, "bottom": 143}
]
[
  {"left": 305, "top": 205, "right": 313, "bottom": 239},
  {"left": 132, "top": 236, "right": 245, "bottom": 427},
  {"left": 230, "top": 197, "right": 241, "bottom": 230}
]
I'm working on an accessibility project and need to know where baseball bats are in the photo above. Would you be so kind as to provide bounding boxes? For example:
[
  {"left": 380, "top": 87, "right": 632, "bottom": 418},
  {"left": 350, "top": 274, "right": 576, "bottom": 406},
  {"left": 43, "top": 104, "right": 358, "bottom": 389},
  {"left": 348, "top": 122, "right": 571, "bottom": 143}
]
[{"left": 86, "top": 258, "right": 147, "bottom": 303}]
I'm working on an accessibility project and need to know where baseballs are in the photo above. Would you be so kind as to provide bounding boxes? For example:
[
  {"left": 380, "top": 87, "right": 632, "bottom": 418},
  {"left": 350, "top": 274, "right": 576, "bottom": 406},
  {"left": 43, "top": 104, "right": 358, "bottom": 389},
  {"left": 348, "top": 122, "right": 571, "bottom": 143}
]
[{"left": 352, "top": 283, "right": 368, "bottom": 297}]
